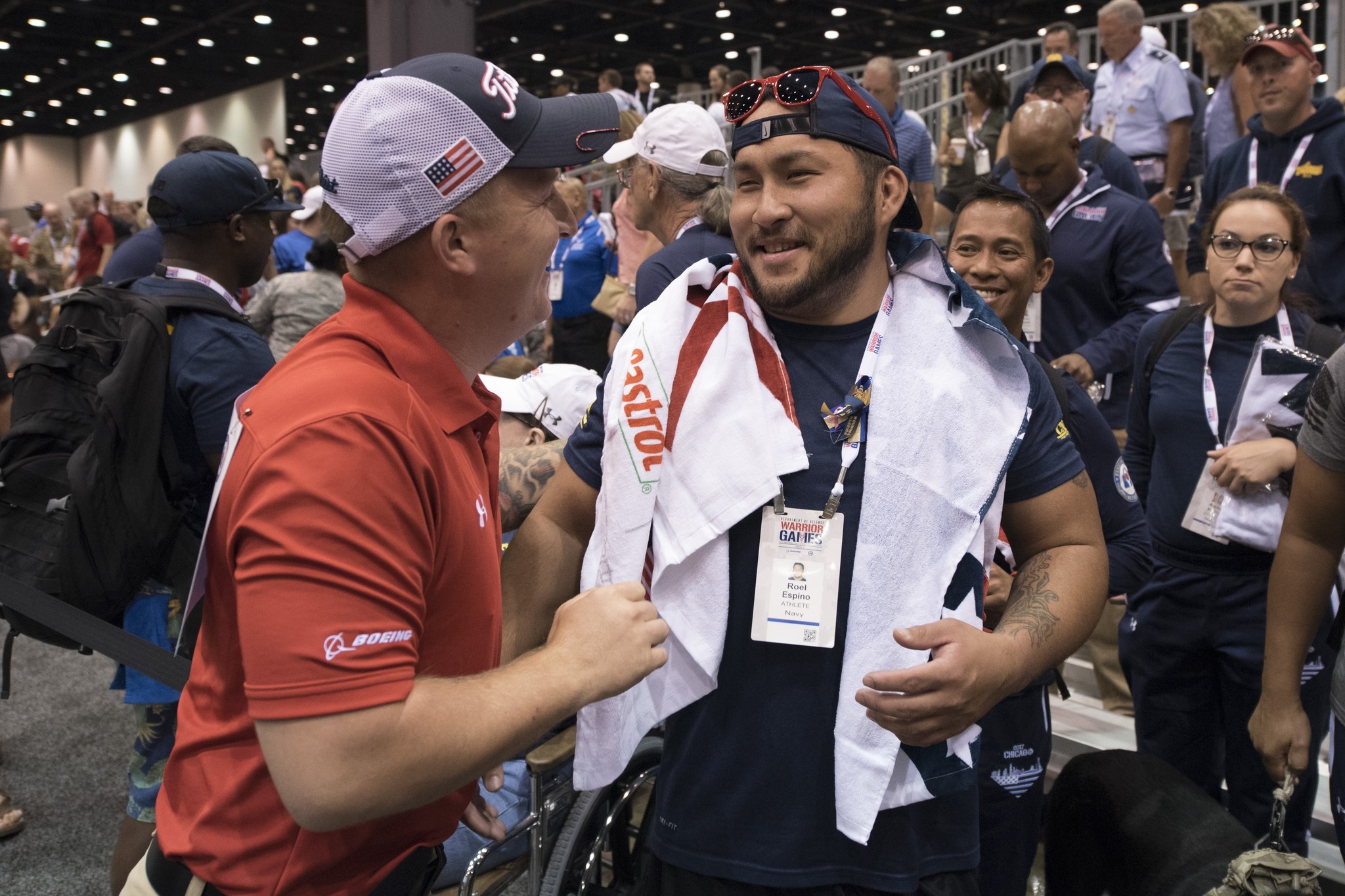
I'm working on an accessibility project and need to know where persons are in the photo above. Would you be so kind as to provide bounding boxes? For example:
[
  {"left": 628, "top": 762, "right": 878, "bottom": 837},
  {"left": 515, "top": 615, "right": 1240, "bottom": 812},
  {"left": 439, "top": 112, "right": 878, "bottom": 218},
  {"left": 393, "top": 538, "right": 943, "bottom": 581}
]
[
  {"left": 937, "top": 175, "right": 1152, "bottom": 896},
  {"left": 494, "top": 66, "right": 1110, "bottom": 895},
  {"left": 1119, "top": 185, "right": 1332, "bottom": 866},
  {"left": 111, "top": 151, "right": 306, "bottom": 895},
  {"left": 0, "top": 0, "right": 1345, "bottom": 842},
  {"left": 113, "top": 50, "right": 667, "bottom": 896},
  {"left": 1248, "top": 325, "right": 1345, "bottom": 896}
]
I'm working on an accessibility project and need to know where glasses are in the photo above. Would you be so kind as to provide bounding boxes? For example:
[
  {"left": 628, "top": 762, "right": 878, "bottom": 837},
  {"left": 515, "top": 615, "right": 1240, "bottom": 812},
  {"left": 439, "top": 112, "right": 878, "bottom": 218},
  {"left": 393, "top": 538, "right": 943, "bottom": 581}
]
[
  {"left": 1209, "top": 234, "right": 1294, "bottom": 262},
  {"left": 722, "top": 65, "right": 897, "bottom": 158},
  {"left": 1243, "top": 26, "right": 1314, "bottom": 58},
  {"left": 1031, "top": 84, "right": 1079, "bottom": 98},
  {"left": 222, "top": 178, "right": 284, "bottom": 228}
]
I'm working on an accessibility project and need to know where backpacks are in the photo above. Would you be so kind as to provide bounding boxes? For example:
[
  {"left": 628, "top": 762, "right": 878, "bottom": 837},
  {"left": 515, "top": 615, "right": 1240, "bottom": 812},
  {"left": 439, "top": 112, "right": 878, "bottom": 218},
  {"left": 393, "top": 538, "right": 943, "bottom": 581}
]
[
  {"left": 86, "top": 211, "right": 133, "bottom": 254},
  {"left": 1, "top": 275, "right": 259, "bottom": 656}
]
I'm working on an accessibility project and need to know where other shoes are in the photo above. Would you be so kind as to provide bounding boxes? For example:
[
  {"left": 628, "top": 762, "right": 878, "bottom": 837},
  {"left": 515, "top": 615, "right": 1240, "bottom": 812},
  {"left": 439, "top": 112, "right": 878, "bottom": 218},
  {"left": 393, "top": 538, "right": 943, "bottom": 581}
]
[{"left": 0, "top": 787, "right": 28, "bottom": 838}]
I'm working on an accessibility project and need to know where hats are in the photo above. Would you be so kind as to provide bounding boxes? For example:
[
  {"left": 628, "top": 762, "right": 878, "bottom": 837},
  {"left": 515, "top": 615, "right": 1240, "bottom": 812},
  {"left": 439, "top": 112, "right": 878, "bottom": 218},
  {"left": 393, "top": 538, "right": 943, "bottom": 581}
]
[
  {"left": 317, "top": 51, "right": 620, "bottom": 266},
  {"left": 24, "top": 202, "right": 43, "bottom": 211},
  {"left": 731, "top": 71, "right": 923, "bottom": 230},
  {"left": 603, "top": 99, "right": 729, "bottom": 178},
  {"left": 291, "top": 185, "right": 324, "bottom": 219},
  {"left": 1240, "top": 23, "right": 1318, "bottom": 66},
  {"left": 1029, "top": 54, "right": 1086, "bottom": 86},
  {"left": 145, "top": 150, "right": 305, "bottom": 236}
]
